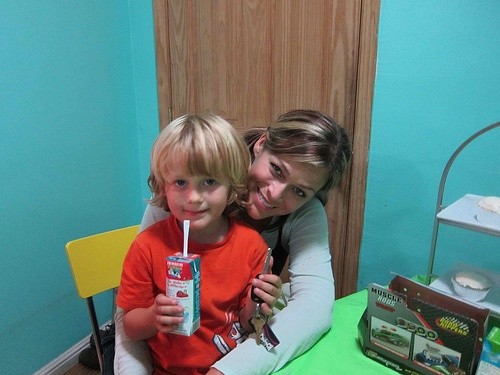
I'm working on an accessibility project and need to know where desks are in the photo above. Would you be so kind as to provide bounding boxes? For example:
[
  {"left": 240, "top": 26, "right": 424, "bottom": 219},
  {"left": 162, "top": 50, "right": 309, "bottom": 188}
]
[{"left": 274, "top": 288, "right": 500, "bottom": 375}]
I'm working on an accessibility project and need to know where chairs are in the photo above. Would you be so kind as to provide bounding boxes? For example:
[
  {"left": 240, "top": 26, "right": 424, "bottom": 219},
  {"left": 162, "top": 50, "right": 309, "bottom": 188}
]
[{"left": 65, "top": 223, "right": 141, "bottom": 375}]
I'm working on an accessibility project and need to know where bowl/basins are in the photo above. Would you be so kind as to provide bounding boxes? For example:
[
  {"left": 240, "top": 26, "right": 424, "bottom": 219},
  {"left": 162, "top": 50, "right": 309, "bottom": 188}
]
[{"left": 451, "top": 269, "right": 491, "bottom": 302}]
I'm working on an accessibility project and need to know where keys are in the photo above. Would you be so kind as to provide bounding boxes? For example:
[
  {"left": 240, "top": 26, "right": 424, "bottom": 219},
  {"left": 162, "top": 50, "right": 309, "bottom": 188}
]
[{"left": 250, "top": 248, "right": 280, "bottom": 352}]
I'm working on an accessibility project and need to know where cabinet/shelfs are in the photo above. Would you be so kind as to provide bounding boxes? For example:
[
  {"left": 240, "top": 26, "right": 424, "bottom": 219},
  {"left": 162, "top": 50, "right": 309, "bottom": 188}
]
[{"left": 426, "top": 121, "right": 500, "bottom": 375}]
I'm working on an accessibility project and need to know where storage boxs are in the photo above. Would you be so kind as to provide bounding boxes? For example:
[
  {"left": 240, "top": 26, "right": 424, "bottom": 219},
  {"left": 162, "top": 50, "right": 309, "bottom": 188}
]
[{"left": 356, "top": 271, "right": 490, "bottom": 375}]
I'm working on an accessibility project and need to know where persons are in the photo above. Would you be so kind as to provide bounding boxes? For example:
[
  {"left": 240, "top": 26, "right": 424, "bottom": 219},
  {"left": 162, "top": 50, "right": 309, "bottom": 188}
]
[
  {"left": 115, "top": 112, "right": 282, "bottom": 375},
  {"left": 113, "top": 110, "right": 352, "bottom": 375}
]
